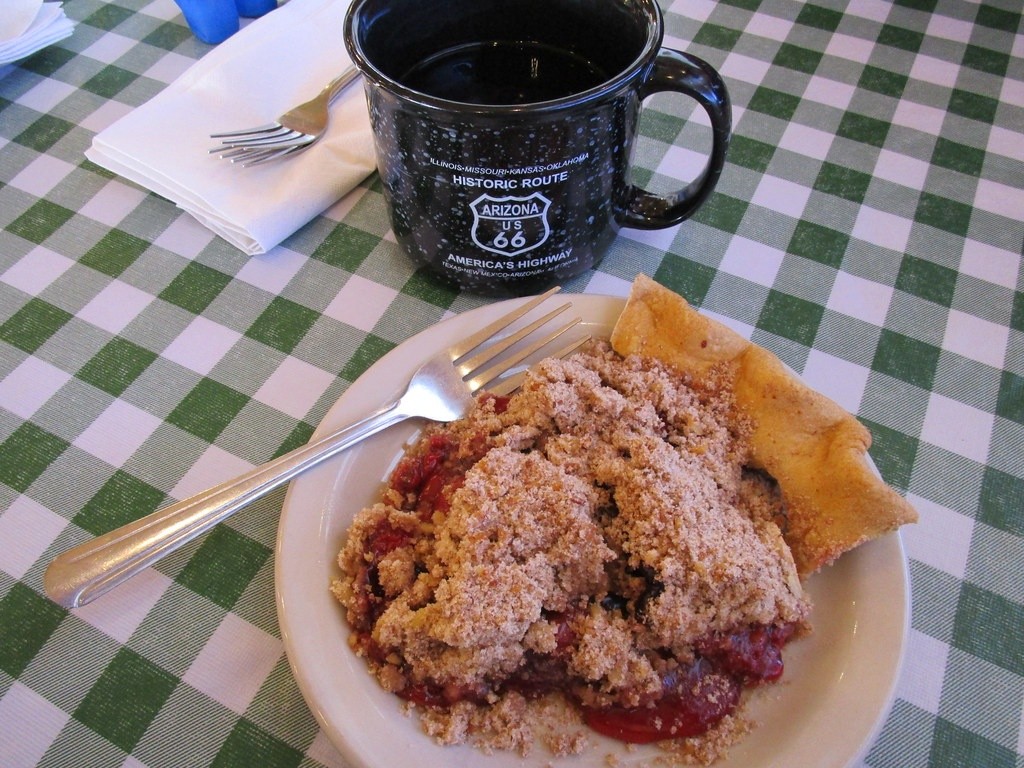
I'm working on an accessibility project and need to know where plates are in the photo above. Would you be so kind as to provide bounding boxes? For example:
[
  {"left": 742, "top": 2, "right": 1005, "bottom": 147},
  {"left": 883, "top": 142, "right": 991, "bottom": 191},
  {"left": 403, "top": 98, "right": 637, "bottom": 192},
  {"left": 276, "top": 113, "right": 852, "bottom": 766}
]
[{"left": 274, "top": 292, "right": 913, "bottom": 768}]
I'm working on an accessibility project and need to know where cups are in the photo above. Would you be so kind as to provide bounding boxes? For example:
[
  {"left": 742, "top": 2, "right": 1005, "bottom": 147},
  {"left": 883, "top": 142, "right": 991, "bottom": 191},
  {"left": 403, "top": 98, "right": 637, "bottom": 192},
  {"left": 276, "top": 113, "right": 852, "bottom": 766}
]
[{"left": 343, "top": 0, "right": 733, "bottom": 298}]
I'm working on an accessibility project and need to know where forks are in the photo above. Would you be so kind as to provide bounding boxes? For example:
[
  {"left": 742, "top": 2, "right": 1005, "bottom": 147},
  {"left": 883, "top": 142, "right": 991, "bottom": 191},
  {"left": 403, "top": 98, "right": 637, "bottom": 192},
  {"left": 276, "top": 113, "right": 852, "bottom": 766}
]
[
  {"left": 209, "top": 64, "right": 360, "bottom": 153},
  {"left": 42, "top": 285, "right": 594, "bottom": 611},
  {"left": 208, "top": 72, "right": 361, "bottom": 169}
]
